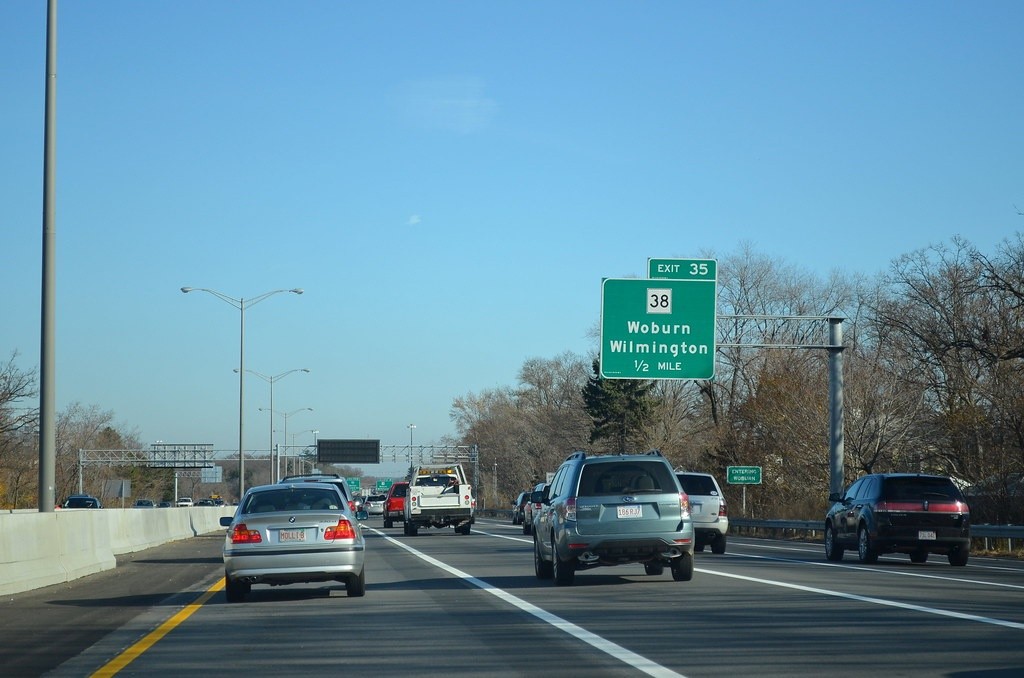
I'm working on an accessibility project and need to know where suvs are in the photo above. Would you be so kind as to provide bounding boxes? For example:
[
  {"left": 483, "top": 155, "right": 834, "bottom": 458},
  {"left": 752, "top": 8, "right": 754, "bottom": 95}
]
[
  {"left": 279, "top": 473, "right": 358, "bottom": 516},
  {"left": 382, "top": 482, "right": 409, "bottom": 529},
  {"left": 529, "top": 450, "right": 696, "bottom": 586}
]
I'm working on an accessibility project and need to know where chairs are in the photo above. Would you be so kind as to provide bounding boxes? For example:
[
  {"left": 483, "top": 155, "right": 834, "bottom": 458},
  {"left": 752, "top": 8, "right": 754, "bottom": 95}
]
[
  {"left": 630, "top": 474, "right": 654, "bottom": 490},
  {"left": 311, "top": 494, "right": 336, "bottom": 510},
  {"left": 593, "top": 474, "right": 617, "bottom": 493},
  {"left": 253, "top": 500, "right": 275, "bottom": 512}
]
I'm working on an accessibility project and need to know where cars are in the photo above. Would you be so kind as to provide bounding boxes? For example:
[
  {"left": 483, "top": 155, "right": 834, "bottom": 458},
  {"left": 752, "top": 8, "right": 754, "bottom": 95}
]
[
  {"left": 60, "top": 494, "right": 224, "bottom": 508},
  {"left": 510, "top": 483, "right": 550, "bottom": 536},
  {"left": 220, "top": 483, "right": 369, "bottom": 603},
  {"left": 825, "top": 474, "right": 971, "bottom": 567},
  {"left": 351, "top": 491, "right": 386, "bottom": 517},
  {"left": 673, "top": 471, "right": 730, "bottom": 554}
]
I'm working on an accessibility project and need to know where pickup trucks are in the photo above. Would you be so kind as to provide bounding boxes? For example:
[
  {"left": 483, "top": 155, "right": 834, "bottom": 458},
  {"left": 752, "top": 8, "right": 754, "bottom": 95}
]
[{"left": 403, "top": 463, "right": 473, "bottom": 536}]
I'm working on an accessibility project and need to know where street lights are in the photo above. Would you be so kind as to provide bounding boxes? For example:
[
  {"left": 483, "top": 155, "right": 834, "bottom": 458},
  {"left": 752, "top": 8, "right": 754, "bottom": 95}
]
[
  {"left": 233, "top": 367, "right": 311, "bottom": 484},
  {"left": 407, "top": 423, "right": 417, "bottom": 470},
  {"left": 181, "top": 286, "right": 304, "bottom": 503},
  {"left": 258, "top": 406, "right": 318, "bottom": 479}
]
